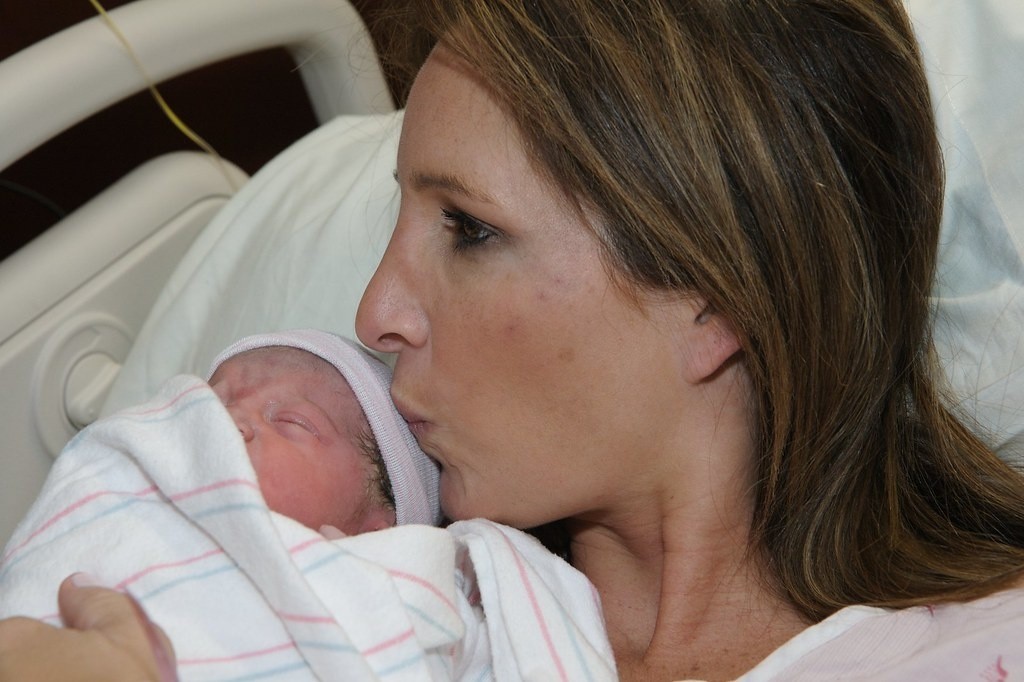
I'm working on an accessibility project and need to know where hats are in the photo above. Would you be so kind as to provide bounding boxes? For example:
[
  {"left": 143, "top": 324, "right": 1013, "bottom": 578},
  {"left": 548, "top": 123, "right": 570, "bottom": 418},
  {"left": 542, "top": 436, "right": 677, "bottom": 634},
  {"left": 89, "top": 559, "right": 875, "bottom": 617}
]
[{"left": 207, "top": 328, "right": 442, "bottom": 527}]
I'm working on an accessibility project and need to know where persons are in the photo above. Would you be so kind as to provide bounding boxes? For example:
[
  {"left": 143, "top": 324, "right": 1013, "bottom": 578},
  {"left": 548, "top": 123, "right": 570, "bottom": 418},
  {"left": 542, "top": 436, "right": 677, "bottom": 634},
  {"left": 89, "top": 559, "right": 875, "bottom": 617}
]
[{"left": 0, "top": 0, "right": 1024, "bottom": 682}]
